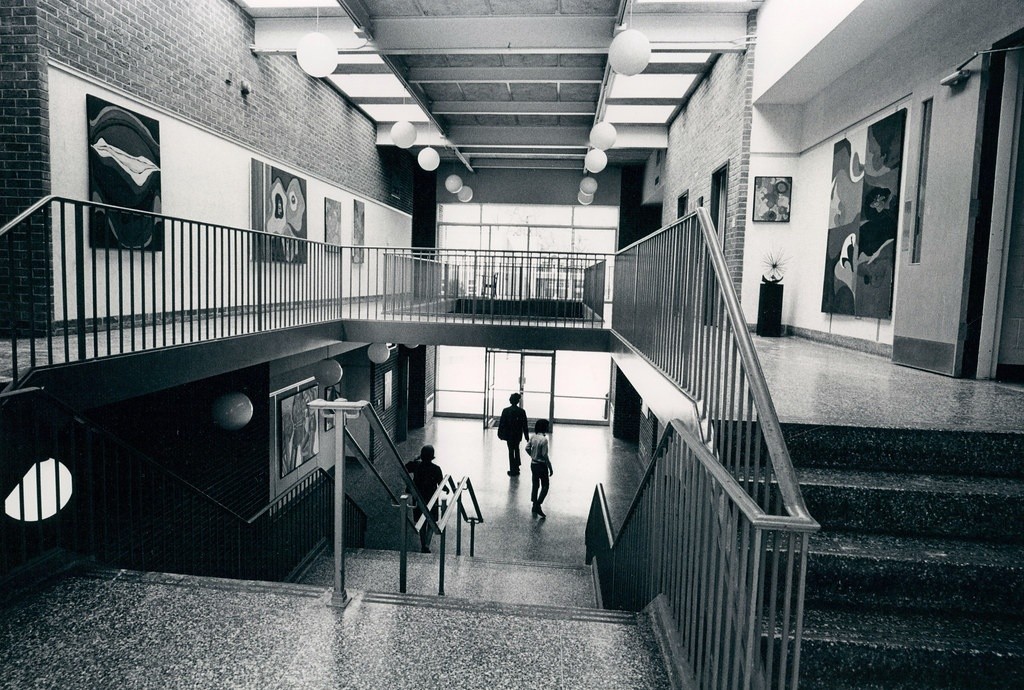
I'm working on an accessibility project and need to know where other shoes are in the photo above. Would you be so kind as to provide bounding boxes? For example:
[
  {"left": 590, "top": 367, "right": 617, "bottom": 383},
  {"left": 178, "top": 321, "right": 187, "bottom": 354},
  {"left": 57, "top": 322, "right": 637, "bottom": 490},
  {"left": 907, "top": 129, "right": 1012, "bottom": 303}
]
[
  {"left": 532, "top": 506, "right": 546, "bottom": 517},
  {"left": 508, "top": 471, "right": 520, "bottom": 475}
]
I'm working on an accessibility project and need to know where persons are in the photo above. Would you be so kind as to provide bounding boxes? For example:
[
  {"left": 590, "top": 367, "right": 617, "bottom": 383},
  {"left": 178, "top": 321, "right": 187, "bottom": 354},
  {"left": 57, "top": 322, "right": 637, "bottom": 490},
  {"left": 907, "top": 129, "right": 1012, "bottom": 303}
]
[
  {"left": 404, "top": 445, "right": 449, "bottom": 553},
  {"left": 525, "top": 419, "right": 553, "bottom": 518},
  {"left": 497, "top": 392, "right": 529, "bottom": 477}
]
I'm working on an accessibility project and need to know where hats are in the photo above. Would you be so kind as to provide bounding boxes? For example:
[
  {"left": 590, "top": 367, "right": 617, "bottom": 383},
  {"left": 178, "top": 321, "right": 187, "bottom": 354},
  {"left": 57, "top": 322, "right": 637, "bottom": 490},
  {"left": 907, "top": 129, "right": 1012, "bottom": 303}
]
[{"left": 420, "top": 445, "right": 435, "bottom": 460}]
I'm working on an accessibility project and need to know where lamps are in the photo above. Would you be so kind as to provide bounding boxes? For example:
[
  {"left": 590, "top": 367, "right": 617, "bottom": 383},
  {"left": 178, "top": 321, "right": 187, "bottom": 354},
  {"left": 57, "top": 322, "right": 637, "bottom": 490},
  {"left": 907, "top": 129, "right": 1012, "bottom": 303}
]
[
  {"left": 580, "top": 171, "right": 598, "bottom": 196},
  {"left": 296, "top": 8, "right": 338, "bottom": 78},
  {"left": 390, "top": 97, "right": 418, "bottom": 148},
  {"left": 367, "top": 342, "right": 391, "bottom": 364},
  {"left": 578, "top": 190, "right": 594, "bottom": 205},
  {"left": 590, "top": 121, "right": 617, "bottom": 151},
  {"left": 444, "top": 158, "right": 463, "bottom": 194},
  {"left": 608, "top": 0, "right": 651, "bottom": 76},
  {"left": 211, "top": 390, "right": 254, "bottom": 431},
  {"left": 417, "top": 122, "right": 440, "bottom": 171},
  {"left": 314, "top": 346, "right": 344, "bottom": 386},
  {"left": 457, "top": 186, "right": 473, "bottom": 202},
  {"left": 5, "top": 456, "right": 72, "bottom": 522},
  {"left": 405, "top": 344, "right": 419, "bottom": 348},
  {"left": 584, "top": 147, "right": 608, "bottom": 173}
]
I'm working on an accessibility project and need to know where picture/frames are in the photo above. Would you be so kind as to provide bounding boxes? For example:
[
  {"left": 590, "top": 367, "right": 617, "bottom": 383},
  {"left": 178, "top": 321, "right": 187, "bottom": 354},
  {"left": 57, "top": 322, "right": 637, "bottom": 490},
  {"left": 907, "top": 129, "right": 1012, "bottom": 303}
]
[
  {"left": 753, "top": 176, "right": 792, "bottom": 223},
  {"left": 325, "top": 383, "right": 342, "bottom": 431},
  {"left": 279, "top": 385, "right": 320, "bottom": 478}
]
